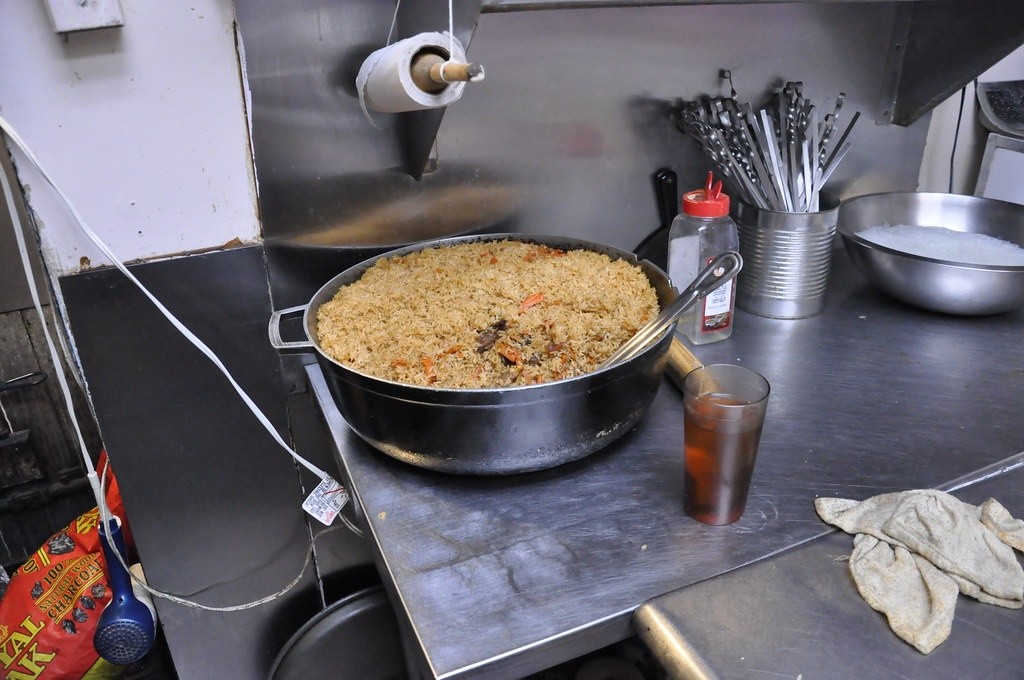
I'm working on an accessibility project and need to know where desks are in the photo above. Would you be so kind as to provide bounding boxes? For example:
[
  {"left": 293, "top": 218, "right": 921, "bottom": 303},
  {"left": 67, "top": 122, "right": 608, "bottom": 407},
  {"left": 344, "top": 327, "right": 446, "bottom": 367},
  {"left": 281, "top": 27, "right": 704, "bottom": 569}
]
[{"left": 633, "top": 462, "right": 1024, "bottom": 680}]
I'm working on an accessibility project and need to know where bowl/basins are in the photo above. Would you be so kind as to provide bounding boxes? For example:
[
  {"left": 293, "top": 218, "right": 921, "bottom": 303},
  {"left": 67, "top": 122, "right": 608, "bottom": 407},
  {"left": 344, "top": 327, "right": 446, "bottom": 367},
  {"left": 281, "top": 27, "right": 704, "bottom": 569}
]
[{"left": 836, "top": 192, "right": 1024, "bottom": 317}]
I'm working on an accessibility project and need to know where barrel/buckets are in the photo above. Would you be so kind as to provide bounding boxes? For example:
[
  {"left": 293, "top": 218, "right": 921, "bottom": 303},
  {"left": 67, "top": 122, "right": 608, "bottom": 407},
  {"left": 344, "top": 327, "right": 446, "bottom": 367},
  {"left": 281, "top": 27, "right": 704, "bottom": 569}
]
[{"left": 734, "top": 189, "right": 840, "bottom": 322}]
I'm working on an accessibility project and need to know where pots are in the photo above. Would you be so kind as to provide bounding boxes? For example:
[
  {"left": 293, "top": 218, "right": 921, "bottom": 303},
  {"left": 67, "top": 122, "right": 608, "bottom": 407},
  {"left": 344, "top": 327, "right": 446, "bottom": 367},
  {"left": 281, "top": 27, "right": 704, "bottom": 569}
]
[{"left": 268, "top": 232, "right": 680, "bottom": 477}]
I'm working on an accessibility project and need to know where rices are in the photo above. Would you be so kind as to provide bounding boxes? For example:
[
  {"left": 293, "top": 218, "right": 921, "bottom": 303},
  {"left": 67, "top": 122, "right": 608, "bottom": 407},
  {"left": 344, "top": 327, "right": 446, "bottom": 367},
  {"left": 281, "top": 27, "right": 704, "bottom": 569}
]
[{"left": 313, "top": 237, "right": 659, "bottom": 390}]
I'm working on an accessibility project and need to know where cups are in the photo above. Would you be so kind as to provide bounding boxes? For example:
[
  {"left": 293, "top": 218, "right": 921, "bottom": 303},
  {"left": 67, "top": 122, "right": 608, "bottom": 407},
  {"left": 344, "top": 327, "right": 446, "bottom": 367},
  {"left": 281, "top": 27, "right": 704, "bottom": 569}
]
[{"left": 682, "top": 363, "right": 771, "bottom": 526}]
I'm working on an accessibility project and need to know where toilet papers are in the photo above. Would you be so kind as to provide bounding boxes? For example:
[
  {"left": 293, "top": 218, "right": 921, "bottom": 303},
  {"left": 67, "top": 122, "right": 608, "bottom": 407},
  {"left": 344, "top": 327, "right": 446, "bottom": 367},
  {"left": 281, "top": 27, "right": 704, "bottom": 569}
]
[{"left": 356, "top": 31, "right": 469, "bottom": 131}]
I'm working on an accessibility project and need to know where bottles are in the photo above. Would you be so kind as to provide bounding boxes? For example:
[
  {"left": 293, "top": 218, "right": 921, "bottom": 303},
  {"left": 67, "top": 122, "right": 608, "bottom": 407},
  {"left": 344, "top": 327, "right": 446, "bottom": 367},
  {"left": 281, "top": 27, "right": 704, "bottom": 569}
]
[{"left": 669, "top": 171, "right": 741, "bottom": 345}]
